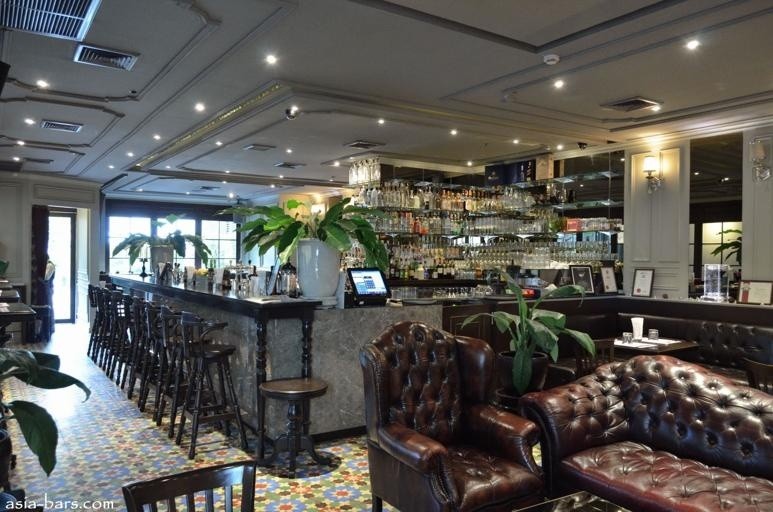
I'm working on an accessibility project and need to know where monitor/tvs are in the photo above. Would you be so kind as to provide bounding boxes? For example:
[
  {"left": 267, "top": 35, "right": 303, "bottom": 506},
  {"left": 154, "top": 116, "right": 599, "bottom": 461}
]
[{"left": 346, "top": 268, "right": 391, "bottom": 306}]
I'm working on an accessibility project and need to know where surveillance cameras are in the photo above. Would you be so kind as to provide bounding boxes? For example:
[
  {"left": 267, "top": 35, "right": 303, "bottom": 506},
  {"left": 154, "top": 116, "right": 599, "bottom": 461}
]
[
  {"left": 285, "top": 108, "right": 298, "bottom": 121},
  {"left": 578, "top": 143, "right": 587, "bottom": 151}
]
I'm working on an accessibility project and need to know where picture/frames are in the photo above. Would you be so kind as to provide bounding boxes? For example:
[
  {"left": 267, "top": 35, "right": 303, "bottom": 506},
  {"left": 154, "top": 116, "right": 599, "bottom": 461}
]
[
  {"left": 736, "top": 279, "right": 772, "bottom": 305},
  {"left": 599, "top": 266, "right": 617, "bottom": 293},
  {"left": 569, "top": 265, "right": 596, "bottom": 297},
  {"left": 631, "top": 268, "right": 654, "bottom": 297}
]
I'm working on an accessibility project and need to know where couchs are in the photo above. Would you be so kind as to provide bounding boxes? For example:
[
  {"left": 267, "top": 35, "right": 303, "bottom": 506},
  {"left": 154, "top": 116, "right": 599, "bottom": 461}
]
[{"left": 521, "top": 353, "right": 772, "bottom": 510}]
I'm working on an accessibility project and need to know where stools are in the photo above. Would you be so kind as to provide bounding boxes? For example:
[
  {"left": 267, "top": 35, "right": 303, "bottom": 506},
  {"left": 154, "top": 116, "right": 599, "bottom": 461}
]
[{"left": 260, "top": 376, "right": 331, "bottom": 479}]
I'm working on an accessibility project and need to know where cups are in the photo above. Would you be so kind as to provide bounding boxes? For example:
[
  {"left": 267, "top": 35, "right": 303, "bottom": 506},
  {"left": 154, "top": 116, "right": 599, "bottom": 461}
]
[
  {"left": 464, "top": 241, "right": 608, "bottom": 268},
  {"left": 288, "top": 275, "right": 297, "bottom": 297},
  {"left": 729, "top": 281, "right": 739, "bottom": 301},
  {"left": 622, "top": 332, "right": 632, "bottom": 344},
  {"left": 647, "top": 329, "right": 658, "bottom": 340},
  {"left": 630, "top": 317, "right": 644, "bottom": 340},
  {"left": 741, "top": 286, "right": 749, "bottom": 302}
]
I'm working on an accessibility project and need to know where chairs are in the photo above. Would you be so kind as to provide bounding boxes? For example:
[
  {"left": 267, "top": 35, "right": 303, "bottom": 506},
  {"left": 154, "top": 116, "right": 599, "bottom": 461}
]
[
  {"left": 121, "top": 460, "right": 257, "bottom": 512},
  {"left": 86, "top": 283, "right": 248, "bottom": 459},
  {"left": 574, "top": 338, "right": 613, "bottom": 378},
  {"left": 357, "top": 319, "right": 543, "bottom": 509},
  {"left": 742, "top": 357, "right": 773, "bottom": 395}
]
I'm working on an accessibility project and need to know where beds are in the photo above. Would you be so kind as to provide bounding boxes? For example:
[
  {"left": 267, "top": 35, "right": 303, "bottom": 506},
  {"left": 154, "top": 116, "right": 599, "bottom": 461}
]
[{"left": 615, "top": 335, "right": 700, "bottom": 361}]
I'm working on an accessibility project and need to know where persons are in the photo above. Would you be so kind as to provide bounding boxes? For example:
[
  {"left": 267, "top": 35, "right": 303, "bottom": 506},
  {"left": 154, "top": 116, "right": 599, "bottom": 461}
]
[{"left": 45, "top": 253, "right": 55, "bottom": 334}]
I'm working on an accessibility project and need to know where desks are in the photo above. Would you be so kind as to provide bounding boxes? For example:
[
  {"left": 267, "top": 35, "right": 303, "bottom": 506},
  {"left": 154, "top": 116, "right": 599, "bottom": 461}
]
[{"left": 0, "top": 278, "right": 51, "bottom": 347}]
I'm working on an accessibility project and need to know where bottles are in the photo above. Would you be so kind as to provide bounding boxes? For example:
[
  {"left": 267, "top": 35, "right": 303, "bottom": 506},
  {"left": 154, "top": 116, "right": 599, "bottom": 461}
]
[
  {"left": 526, "top": 161, "right": 533, "bottom": 182},
  {"left": 519, "top": 163, "right": 524, "bottom": 182},
  {"left": 207, "top": 258, "right": 280, "bottom": 297},
  {"left": 173, "top": 262, "right": 181, "bottom": 280},
  {"left": 350, "top": 180, "right": 567, "bottom": 211},
  {"left": 360, "top": 210, "right": 559, "bottom": 235},
  {"left": 279, "top": 256, "right": 296, "bottom": 293},
  {"left": 393, "top": 285, "right": 493, "bottom": 300},
  {"left": 518, "top": 269, "right": 539, "bottom": 288},
  {"left": 346, "top": 158, "right": 381, "bottom": 183},
  {"left": 346, "top": 236, "right": 476, "bottom": 280}
]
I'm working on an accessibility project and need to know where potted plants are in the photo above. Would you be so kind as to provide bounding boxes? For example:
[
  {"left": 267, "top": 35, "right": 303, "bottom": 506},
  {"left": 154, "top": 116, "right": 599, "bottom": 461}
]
[
  {"left": 712, "top": 230, "right": 741, "bottom": 279},
  {"left": 210, "top": 194, "right": 388, "bottom": 300},
  {"left": 112, "top": 213, "right": 211, "bottom": 274},
  {"left": 1, "top": 341, "right": 90, "bottom": 485},
  {"left": 459, "top": 264, "right": 595, "bottom": 390}
]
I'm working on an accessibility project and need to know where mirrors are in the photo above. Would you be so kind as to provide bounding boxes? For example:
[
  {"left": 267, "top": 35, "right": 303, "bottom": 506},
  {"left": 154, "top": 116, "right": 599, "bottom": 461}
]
[{"left": 688, "top": 130, "right": 743, "bottom": 301}]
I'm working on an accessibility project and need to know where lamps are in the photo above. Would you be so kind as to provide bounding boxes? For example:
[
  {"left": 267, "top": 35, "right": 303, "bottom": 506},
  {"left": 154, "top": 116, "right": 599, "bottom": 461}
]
[
  {"left": 641, "top": 155, "right": 664, "bottom": 195},
  {"left": 748, "top": 134, "right": 772, "bottom": 183}
]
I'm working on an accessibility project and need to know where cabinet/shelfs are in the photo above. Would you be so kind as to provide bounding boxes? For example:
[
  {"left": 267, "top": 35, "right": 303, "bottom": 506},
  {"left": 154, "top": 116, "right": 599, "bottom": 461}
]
[{"left": 341, "top": 206, "right": 622, "bottom": 279}]
